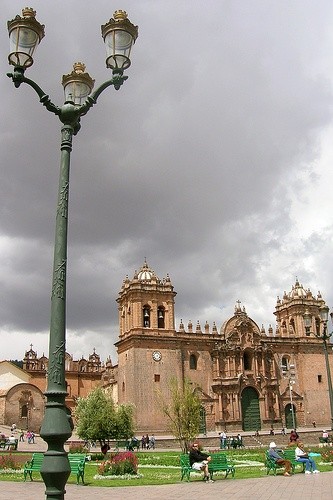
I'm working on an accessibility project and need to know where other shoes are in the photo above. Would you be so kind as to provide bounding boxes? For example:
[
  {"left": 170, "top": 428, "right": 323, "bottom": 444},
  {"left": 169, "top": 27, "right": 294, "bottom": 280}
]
[
  {"left": 284, "top": 472, "right": 292, "bottom": 476},
  {"left": 313, "top": 470, "right": 320, "bottom": 473},
  {"left": 206, "top": 479, "right": 214, "bottom": 483},
  {"left": 204, "top": 474, "right": 208, "bottom": 477},
  {"left": 305, "top": 471, "right": 311, "bottom": 473}
]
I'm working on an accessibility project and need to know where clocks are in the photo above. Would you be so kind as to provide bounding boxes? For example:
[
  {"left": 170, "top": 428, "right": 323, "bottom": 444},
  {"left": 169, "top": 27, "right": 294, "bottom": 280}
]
[{"left": 152, "top": 350, "right": 162, "bottom": 362}]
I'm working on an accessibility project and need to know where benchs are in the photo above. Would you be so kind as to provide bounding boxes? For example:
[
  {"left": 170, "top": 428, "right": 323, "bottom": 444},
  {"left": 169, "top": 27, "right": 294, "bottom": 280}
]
[
  {"left": 264, "top": 450, "right": 316, "bottom": 476},
  {"left": 24, "top": 453, "right": 87, "bottom": 485},
  {"left": 220, "top": 440, "right": 242, "bottom": 449},
  {"left": 2, "top": 442, "right": 15, "bottom": 450},
  {"left": 113, "top": 441, "right": 140, "bottom": 452},
  {"left": 319, "top": 436, "right": 333, "bottom": 443},
  {"left": 179, "top": 454, "right": 235, "bottom": 483}
]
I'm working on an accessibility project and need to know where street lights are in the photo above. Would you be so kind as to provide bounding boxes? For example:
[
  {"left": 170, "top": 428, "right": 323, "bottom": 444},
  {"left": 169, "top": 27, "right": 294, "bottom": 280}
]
[
  {"left": 4, "top": 5, "right": 139, "bottom": 499},
  {"left": 302, "top": 302, "right": 333, "bottom": 420}
]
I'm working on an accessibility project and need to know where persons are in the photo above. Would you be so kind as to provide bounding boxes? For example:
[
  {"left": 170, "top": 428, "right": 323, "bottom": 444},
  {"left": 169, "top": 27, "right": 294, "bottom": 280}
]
[
  {"left": 25, "top": 430, "right": 35, "bottom": 444},
  {"left": 149, "top": 434, "right": 155, "bottom": 450},
  {"left": 0, "top": 432, "right": 5, "bottom": 448},
  {"left": 7, "top": 435, "right": 15, "bottom": 450},
  {"left": 290, "top": 429, "right": 299, "bottom": 442},
  {"left": 141, "top": 435, "right": 146, "bottom": 450},
  {"left": 145, "top": 433, "right": 150, "bottom": 450},
  {"left": 12, "top": 422, "right": 17, "bottom": 434},
  {"left": 269, "top": 442, "right": 292, "bottom": 477},
  {"left": 218, "top": 428, "right": 241, "bottom": 449},
  {"left": 295, "top": 441, "right": 320, "bottom": 474},
  {"left": 19, "top": 429, "right": 24, "bottom": 442},
  {"left": 189, "top": 442, "right": 214, "bottom": 483}
]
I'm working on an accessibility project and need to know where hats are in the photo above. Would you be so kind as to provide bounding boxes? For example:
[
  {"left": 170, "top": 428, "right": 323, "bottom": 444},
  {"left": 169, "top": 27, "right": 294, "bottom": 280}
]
[{"left": 270, "top": 442, "right": 276, "bottom": 447}]
